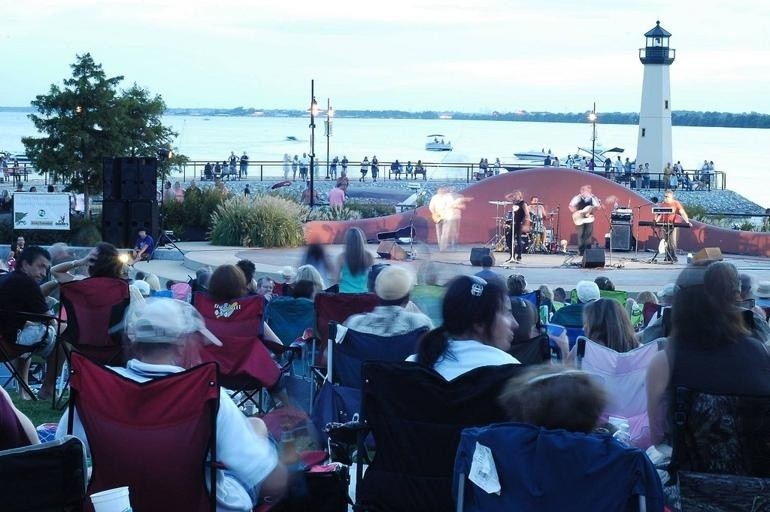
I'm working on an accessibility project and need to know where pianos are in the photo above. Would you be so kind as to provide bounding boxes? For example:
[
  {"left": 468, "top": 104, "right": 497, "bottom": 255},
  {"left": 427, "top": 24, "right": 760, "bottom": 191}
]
[{"left": 639, "top": 221, "right": 692, "bottom": 228}]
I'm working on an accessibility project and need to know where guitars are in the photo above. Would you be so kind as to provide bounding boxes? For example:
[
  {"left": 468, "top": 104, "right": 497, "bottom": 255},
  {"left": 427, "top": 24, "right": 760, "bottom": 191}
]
[
  {"left": 431, "top": 198, "right": 462, "bottom": 224},
  {"left": 572, "top": 195, "right": 618, "bottom": 225}
]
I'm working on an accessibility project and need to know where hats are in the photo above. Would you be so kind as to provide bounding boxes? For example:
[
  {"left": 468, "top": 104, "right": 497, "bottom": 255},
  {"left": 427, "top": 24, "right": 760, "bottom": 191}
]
[
  {"left": 132, "top": 280, "right": 150, "bottom": 296},
  {"left": 277, "top": 266, "right": 297, "bottom": 278},
  {"left": 656, "top": 283, "right": 682, "bottom": 298},
  {"left": 751, "top": 280, "right": 770, "bottom": 298},
  {"left": 133, "top": 300, "right": 225, "bottom": 353},
  {"left": 374, "top": 264, "right": 414, "bottom": 302},
  {"left": 576, "top": 280, "right": 601, "bottom": 305}
]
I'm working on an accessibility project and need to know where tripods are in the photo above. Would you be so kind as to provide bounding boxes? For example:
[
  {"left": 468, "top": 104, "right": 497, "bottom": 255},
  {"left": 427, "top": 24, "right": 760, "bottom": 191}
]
[
  {"left": 147, "top": 158, "right": 184, "bottom": 263},
  {"left": 482, "top": 204, "right": 570, "bottom": 254},
  {"left": 500, "top": 211, "right": 526, "bottom": 266}
]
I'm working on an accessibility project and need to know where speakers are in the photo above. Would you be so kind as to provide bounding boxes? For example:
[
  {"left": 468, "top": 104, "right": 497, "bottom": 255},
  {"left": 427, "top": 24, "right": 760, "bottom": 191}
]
[
  {"left": 582, "top": 249, "right": 605, "bottom": 268},
  {"left": 377, "top": 240, "right": 407, "bottom": 259},
  {"left": 692, "top": 247, "right": 722, "bottom": 265},
  {"left": 103, "top": 156, "right": 159, "bottom": 249},
  {"left": 470, "top": 248, "right": 495, "bottom": 266}
]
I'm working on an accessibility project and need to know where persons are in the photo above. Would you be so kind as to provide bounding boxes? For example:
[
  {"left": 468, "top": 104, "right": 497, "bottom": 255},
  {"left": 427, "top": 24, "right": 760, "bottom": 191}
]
[
  {"left": 391, "top": 159, "right": 402, "bottom": 180},
  {"left": 371, "top": 155, "right": 379, "bottom": 182},
  {"left": 3, "top": 161, "right": 10, "bottom": 181},
  {"left": 336, "top": 171, "right": 350, "bottom": 194},
  {"left": 483, "top": 158, "right": 489, "bottom": 178},
  {"left": 494, "top": 157, "right": 501, "bottom": 175},
  {"left": 301, "top": 180, "right": 319, "bottom": 207},
  {"left": 359, "top": 156, "right": 370, "bottom": 182},
  {"left": 299, "top": 152, "right": 309, "bottom": 182},
  {"left": 13, "top": 160, "right": 21, "bottom": 181},
  {"left": 544, "top": 155, "right": 551, "bottom": 165},
  {"left": 429, "top": 186, "right": 465, "bottom": 253},
  {"left": 503, "top": 189, "right": 529, "bottom": 263},
  {"left": 341, "top": 155, "right": 348, "bottom": 176},
  {"left": 160, "top": 178, "right": 227, "bottom": 206},
  {"left": 405, "top": 160, "right": 414, "bottom": 180},
  {"left": 200, "top": 151, "right": 249, "bottom": 180},
  {"left": 329, "top": 156, "right": 339, "bottom": 179},
  {"left": 480, "top": 158, "right": 484, "bottom": 177},
  {"left": 328, "top": 182, "right": 345, "bottom": 206},
  {"left": 658, "top": 189, "right": 693, "bottom": 261},
  {"left": 526, "top": 196, "right": 552, "bottom": 251},
  {"left": 566, "top": 154, "right": 716, "bottom": 192},
  {"left": 568, "top": 185, "right": 606, "bottom": 255},
  {"left": 0, "top": 181, "right": 55, "bottom": 216},
  {"left": 414, "top": 159, "right": 426, "bottom": 180},
  {"left": 291, "top": 154, "right": 299, "bottom": 181},
  {"left": 552, "top": 156, "right": 560, "bottom": 167}
]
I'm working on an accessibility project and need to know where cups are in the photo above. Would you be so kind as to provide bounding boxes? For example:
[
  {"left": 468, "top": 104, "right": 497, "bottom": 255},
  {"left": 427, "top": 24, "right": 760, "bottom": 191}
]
[
  {"left": 280, "top": 430, "right": 297, "bottom": 463},
  {"left": 89, "top": 485, "right": 131, "bottom": 512}
]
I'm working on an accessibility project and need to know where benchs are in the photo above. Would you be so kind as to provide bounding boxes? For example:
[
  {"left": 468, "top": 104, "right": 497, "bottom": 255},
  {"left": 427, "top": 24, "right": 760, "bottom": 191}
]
[
  {"left": 201, "top": 170, "right": 241, "bottom": 181},
  {"left": 389, "top": 169, "right": 426, "bottom": 179}
]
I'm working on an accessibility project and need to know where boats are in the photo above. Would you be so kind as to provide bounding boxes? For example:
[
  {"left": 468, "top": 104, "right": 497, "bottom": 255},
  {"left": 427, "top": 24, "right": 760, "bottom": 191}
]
[
  {"left": 425, "top": 136, "right": 453, "bottom": 151},
  {"left": 499, "top": 144, "right": 625, "bottom": 175},
  {"left": 514, "top": 151, "right": 556, "bottom": 160}
]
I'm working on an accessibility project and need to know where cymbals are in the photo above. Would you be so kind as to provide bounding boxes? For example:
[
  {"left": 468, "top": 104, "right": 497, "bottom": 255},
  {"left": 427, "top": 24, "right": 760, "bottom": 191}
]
[
  {"left": 530, "top": 202, "right": 544, "bottom": 205},
  {"left": 488, "top": 200, "right": 511, "bottom": 206}
]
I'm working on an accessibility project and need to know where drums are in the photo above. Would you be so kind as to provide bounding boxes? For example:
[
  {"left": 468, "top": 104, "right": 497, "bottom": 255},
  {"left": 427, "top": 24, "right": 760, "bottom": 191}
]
[
  {"left": 530, "top": 230, "right": 545, "bottom": 246},
  {"left": 506, "top": 211, "right": 512, "bottom": 223}
]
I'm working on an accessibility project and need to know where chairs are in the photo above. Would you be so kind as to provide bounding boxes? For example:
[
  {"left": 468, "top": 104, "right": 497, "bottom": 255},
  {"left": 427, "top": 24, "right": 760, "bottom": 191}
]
[{"left": 0, "top": 256, "right": 770, "bottom": 512}]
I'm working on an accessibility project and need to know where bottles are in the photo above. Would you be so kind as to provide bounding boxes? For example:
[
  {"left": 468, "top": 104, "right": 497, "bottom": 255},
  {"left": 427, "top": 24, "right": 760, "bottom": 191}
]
[{"left": 687, "top": 253, "right": 693, "bottom": 265}]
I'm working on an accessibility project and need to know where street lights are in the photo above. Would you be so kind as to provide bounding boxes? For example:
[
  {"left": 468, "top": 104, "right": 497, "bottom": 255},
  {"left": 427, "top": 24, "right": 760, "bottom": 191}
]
[
  {"left": 307, "top": 79, "right": 321, "bottom": 206},
  {"left": 589, "top": 103, "right": 600, "bottom": 170},
  {"left": 323, "top": 95, "right": 335, "bottom": 179}
]
